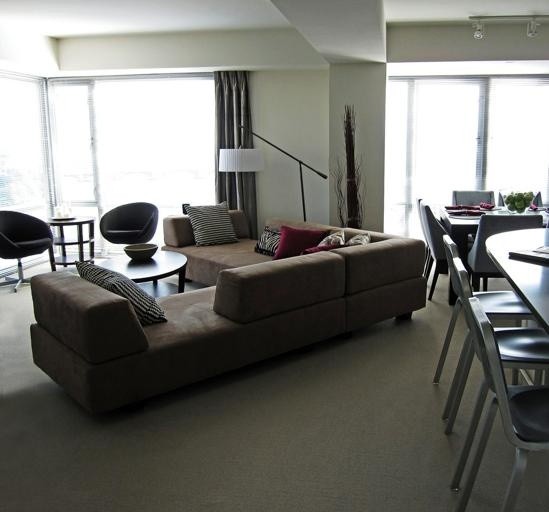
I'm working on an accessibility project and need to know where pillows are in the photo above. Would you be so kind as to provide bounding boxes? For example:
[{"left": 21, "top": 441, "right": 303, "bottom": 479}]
[
  {"left": 253, "top": 223, "right": 371, "bottom": 261},
  {"left": 182, "top": 201, "right": 241, "bottom": 247},
  {"left": 72, "top": 260, "right": 168, "bottom": 327}
]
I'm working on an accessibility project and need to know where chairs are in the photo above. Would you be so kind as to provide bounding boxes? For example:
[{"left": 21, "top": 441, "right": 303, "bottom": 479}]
[
  {"left": 0, "top": 208, "right": 55, "bottom": 293},
  {"left": 99, "top": 201, "right": 159, "bottom": 245}
]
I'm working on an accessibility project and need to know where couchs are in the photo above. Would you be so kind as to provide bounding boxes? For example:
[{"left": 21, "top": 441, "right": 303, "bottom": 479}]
[{"left": 28, "top": 207, "right": 429, "bottom": 421}]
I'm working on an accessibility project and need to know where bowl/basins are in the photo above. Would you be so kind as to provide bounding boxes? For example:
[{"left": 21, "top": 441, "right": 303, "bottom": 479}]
[{"left": 124, "top": 244, "right": 158, "bottom": 260}]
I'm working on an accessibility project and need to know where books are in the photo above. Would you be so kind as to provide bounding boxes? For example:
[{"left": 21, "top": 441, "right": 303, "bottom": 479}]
[{"left": 508, "top": 245, "right": 549, "bottom": 264}]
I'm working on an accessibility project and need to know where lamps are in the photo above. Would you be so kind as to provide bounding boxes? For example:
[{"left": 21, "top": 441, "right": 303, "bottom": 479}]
[
  {"left": 217, "top": 125, "right": 329, "bottom": 222},
  {"left": 473, "top": 16, "right": 542, "bottom": 41}
]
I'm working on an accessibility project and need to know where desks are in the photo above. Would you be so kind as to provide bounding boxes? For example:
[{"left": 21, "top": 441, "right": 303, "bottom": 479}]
[{"left": 92, "top": 250, "right": 188, "bottom": 293}]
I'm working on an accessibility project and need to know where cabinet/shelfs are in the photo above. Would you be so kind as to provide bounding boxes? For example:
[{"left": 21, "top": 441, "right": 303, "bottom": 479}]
[{"left": 44, "top": 217, "right": 96, "bottom": 274}]
